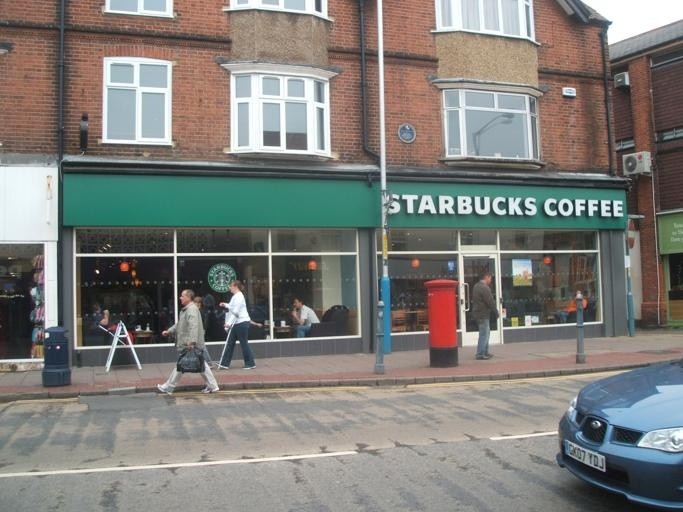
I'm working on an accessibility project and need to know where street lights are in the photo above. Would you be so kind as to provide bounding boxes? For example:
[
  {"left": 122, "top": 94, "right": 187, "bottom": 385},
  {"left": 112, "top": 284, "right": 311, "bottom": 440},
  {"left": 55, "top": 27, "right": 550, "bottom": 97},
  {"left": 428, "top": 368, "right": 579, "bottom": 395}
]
[{"left": 621, "top": 214, "right": 645, "bottom": 338}]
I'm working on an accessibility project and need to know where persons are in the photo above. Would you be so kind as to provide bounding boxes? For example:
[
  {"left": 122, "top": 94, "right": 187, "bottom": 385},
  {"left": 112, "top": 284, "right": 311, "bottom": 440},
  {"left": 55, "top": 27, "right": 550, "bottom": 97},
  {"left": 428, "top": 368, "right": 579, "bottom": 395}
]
[
  {"left": 194, "top": 297, "right": 203, "bottom": 312},
  {"left": 90, "top": 300, "right": 109, "bottom": 331},
  {"left": 155, "top": 288, "right": 218, "bottom": 397},
  {"left": 216, "top": 280, "right": 256, "bottom": 372},
  {"left": 554, "top": 287, "right": 587, "bottom": 324},
  {"left": 289, "top": 295, "right": 320, "bottom": 338},
  {"left": 469, "top": 270, "right": 499, "bottom": 362},
  {"left": 223, "top": 311, "right": 263, "bottom": 334}
]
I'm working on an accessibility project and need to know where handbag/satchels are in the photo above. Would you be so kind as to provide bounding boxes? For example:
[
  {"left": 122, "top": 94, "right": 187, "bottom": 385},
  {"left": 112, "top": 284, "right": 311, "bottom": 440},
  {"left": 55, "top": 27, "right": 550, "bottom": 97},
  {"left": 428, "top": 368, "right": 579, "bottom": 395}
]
[{"left": 177, "top": 349, "right": 204, "bottom": 372}]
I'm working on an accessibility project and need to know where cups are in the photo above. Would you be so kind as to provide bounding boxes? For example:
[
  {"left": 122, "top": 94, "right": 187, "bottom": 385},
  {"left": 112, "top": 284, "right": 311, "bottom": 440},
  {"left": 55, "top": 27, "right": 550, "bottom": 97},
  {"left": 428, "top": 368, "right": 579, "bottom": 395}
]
[
  {"left": 280, "top": 320, "right": 285, "bottom": 327},
  {"left": 136, "top": 325, "right": 140, "bottom": 330},
  {"left": 264, "top": 320, "right": 268, "bottom": 325}
]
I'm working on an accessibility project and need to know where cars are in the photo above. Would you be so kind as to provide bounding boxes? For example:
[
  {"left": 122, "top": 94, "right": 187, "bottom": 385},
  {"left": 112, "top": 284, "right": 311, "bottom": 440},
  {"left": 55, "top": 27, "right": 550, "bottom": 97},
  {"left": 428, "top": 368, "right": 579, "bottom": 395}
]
[
  {"left": 554, "top": 356, "right": 683, "bottom": 511},
  {"left": 522, "top": 279, "right": 598, "bottom": 325}
]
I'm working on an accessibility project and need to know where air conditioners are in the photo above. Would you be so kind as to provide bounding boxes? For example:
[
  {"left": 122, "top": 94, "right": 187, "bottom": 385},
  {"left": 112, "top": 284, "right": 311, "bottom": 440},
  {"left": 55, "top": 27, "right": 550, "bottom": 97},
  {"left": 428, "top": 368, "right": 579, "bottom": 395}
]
[
  {"left": 622, "top": 150, "right": 651, "bottom": 175},
  {"left": 614, "top": 72, "right": 629, "bottom": 89}
]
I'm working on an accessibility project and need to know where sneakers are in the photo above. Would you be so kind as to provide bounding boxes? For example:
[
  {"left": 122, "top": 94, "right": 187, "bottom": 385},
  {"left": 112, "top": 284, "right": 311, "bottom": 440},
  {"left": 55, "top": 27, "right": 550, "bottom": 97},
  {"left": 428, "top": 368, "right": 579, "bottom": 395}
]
[
  {"left": 216, "top": 362, "right": 229, "bottom": 369},
  {"left": 477, "top": 353, "right": 493, "bottom": 359},
  {"left": 157, "top": 382, "right": 172, "bottom": 395},
  {"left": 203, "top": 385, "right": 220, "bottom": 393},
  {"left": 242, "top": 366, "right": 256, "bottom": 369}
]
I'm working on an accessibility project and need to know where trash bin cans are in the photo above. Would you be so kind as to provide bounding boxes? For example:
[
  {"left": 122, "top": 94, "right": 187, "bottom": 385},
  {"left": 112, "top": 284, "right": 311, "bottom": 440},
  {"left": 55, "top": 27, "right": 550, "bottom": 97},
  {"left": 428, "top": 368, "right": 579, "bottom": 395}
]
[{"left": 42, "top": 327, "right": 70, "bottom": 386}]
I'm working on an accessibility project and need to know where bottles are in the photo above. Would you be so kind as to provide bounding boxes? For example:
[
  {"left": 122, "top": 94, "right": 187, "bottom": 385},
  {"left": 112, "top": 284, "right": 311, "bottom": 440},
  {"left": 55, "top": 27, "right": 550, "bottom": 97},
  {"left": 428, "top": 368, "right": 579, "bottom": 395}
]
[{"left": 146, "top": 323, "right": 150, "bottom": 331}]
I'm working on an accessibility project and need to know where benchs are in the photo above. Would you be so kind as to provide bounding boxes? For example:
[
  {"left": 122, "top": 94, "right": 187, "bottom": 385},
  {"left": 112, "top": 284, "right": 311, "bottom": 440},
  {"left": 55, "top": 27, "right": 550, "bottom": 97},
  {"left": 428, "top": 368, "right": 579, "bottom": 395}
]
[{"left": 392, "top": 308, "right": 429, "bottom": 333}]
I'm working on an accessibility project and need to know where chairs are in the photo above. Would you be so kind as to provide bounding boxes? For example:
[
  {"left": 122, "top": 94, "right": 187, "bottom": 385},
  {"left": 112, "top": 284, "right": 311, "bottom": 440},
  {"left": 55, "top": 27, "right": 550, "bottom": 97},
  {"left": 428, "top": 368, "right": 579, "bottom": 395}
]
[
  {"left": 306, "top": 306, "right": 336, "bottom": 338},
  {"left": 567, "top": 304, "right": 595, "bottom": 322},
  {"left": 249, "top": 325, "right": 266, "bottom": 340},
  {"left": 322, "top": 305, "right": 349, "bottom": 336}
]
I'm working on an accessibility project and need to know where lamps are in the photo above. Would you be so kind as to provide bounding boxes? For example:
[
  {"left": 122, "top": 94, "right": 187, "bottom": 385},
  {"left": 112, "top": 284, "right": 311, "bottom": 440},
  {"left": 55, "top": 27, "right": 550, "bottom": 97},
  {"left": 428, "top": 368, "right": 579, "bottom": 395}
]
[
  {"left": 411, "top": 255, "right": 420, "bottom": 267},
  {"left": 544, "top": 256, "right": 552, "bottom": 264},
  {"left": 120, "top": 257, "right": 129, "bottom": 272},
  {"left": 308, "top": 256, "right": 318, "bottom": 271}
]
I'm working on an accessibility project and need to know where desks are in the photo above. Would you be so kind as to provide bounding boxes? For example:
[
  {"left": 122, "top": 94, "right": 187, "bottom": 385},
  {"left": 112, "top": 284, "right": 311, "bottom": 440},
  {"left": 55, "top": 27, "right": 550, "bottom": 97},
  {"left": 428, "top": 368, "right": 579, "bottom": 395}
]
[
  {"left": 130, "top": 331, "right": 155, "bottom": 344},
  {"left": 267, "top": 326, "right": 294, "bottom": 338}
]
